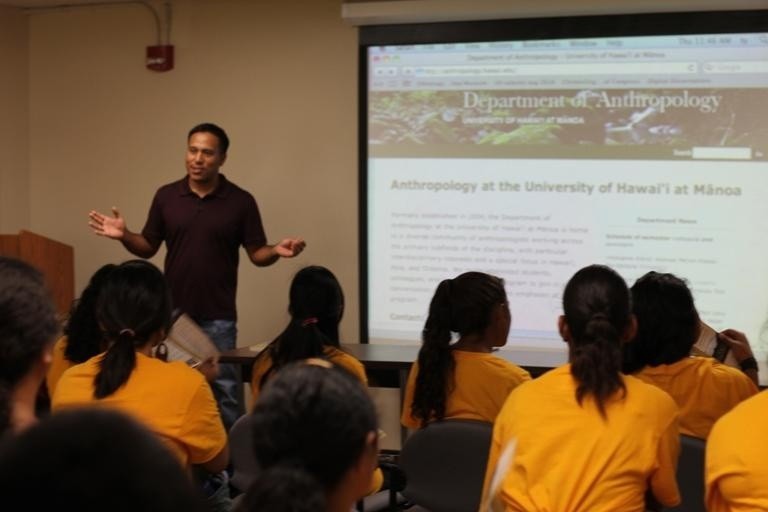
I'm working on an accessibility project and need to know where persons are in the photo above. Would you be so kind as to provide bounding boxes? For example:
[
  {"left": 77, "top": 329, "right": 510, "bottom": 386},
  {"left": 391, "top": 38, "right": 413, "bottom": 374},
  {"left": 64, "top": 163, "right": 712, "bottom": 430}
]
[{"left": 89, "top": 124, "right": 305, "bottom": 351}]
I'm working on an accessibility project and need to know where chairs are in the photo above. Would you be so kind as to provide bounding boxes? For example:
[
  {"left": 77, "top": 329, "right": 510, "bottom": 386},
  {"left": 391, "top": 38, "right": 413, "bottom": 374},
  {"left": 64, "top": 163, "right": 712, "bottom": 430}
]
[{"left": 226, "top": 411, "right": 708, "bottom": 511}]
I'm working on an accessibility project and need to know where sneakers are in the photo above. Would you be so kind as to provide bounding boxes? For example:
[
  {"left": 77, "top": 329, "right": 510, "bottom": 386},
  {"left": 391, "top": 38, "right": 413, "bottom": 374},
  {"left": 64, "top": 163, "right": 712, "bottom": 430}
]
[{"left": 203, "top": 471, "right": 228, "bottom": 499}]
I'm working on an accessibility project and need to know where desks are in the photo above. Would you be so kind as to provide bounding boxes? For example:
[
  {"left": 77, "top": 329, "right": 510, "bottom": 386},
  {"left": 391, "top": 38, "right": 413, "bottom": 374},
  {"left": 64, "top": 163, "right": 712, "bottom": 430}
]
[{"left": 217, "top": 340, "right": 571, "bottom": 446}]
